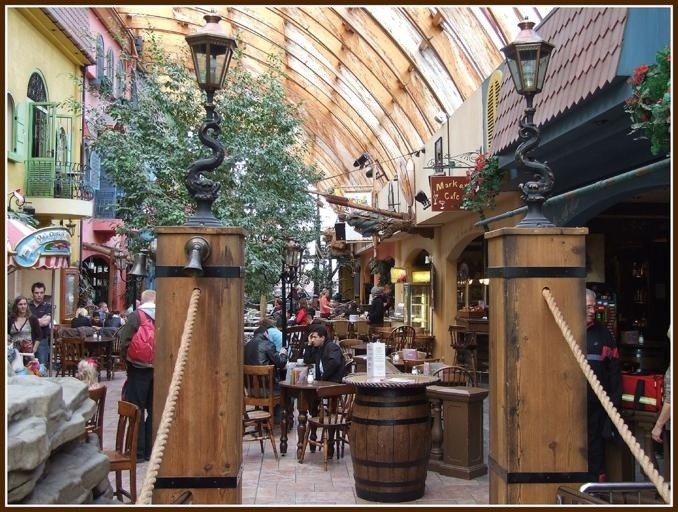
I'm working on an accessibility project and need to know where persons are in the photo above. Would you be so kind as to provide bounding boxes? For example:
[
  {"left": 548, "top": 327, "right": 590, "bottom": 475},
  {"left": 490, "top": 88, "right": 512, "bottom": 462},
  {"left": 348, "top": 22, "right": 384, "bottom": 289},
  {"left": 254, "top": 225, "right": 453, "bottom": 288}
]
[
  {"left": 244, "top": 287, "right": 385, "bottom": 420},
  {"left": 586, "top": 288, "right": 622, "bottom": 483},
  {"left": 8, "top": 281, "right": 156, "bottom": 462},
  {"left": 651, "top": 323, "right": 670, "bottom": 446}
]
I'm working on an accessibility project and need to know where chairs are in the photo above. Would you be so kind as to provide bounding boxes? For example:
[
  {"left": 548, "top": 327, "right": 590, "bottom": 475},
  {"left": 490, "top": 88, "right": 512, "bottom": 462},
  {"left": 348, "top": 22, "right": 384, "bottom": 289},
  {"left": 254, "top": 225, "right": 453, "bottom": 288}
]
[{"left": 39, "top": 310, "right": 477, "bottom": 503}]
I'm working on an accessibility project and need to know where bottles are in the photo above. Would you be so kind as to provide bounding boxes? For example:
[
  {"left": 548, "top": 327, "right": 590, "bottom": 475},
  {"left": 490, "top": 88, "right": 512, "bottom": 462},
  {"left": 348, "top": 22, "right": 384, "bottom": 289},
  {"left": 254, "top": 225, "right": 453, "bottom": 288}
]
[
  {"left": 395, "top": 352, "right": 400, "bottom": 361},
  {"left": 411, "top": 365, "right": 418, "bottom": 375},
  {"left": 307, "top": 369, "right": 314, "bottom": 384},
  {"left": 93, "top": 332, "right": 97, "bottom": 339}
]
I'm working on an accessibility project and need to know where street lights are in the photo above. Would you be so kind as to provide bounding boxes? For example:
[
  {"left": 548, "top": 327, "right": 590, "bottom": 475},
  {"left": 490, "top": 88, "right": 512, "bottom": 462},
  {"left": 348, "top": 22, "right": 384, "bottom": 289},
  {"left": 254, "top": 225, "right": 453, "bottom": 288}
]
[
  {"left": 497, "top": 17, "right": 562, "bottom": 229},
  {"left": 114, "top": 247, "right": 151, "bottom": 314},
  {"left": 280, "top": 231, "right": 305, "bottom": 350},
  {"left": 182, "top": 14, "right": 237, "bottom": 231}
]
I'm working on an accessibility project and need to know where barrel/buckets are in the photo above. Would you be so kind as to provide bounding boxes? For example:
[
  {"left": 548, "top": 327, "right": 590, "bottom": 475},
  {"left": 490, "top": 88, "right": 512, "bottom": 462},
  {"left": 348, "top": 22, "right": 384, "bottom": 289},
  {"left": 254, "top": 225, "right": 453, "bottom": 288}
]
[{"left": 348, "top": 388, "right": 433, "bottom": 503}]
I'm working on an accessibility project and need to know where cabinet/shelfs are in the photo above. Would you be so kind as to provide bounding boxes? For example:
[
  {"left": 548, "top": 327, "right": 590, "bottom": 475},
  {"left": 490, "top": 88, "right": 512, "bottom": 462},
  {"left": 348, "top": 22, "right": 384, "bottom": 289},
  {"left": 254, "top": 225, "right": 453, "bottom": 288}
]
[{"left": 607, "top": 241, "right": 671, "bottom": 354}]
